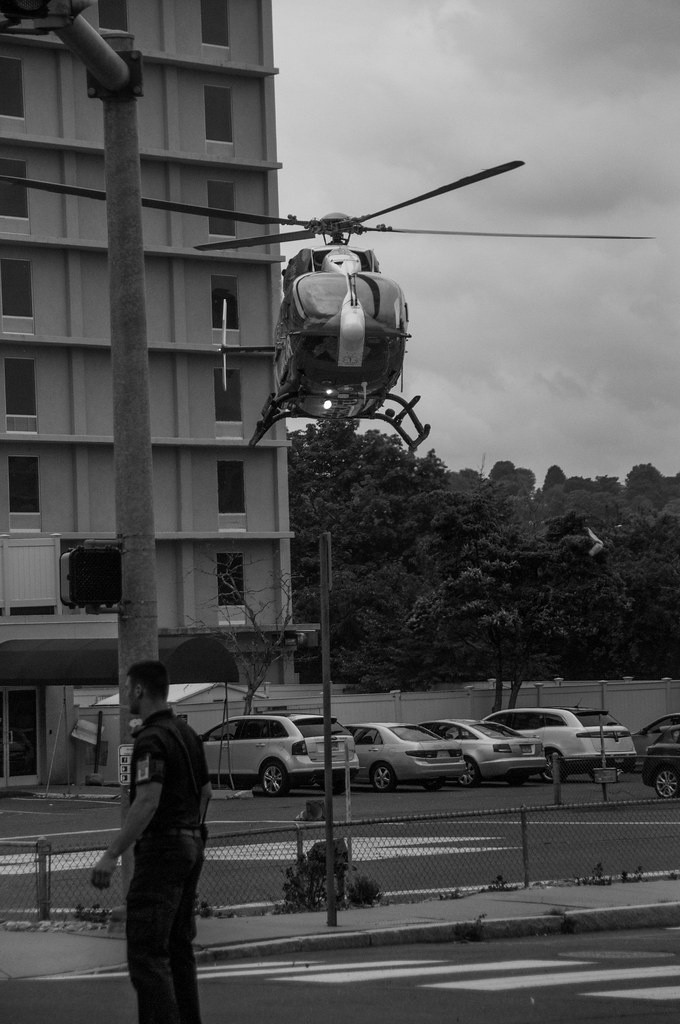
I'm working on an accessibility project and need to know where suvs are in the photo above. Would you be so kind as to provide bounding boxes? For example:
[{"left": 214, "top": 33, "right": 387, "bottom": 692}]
[
  {"left": 195, "top": 712, "right": 359, "bottom": 799},
  {"left": 480, "top": 704, "right": 638, "bottom": 782}
]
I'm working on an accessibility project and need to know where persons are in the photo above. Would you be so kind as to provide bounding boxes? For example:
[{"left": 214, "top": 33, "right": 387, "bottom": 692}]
[{"left": 88, "top": 658, "right": 212, "bottom": 1024}]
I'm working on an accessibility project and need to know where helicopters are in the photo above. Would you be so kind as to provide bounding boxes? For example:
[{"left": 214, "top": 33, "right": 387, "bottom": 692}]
[{"left": 0, "top": 158, "right": 655, "bottom": 467}]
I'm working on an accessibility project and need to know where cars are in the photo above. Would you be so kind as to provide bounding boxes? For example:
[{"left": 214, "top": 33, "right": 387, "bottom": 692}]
[
  {"left": 339, "top": 722, "right": 467, "bottom": 794},
  {"left": 417, "top": 717, "right": 548, "bottom": 788},
  {"left": 640, "top": 722, "right": 680, "bottom": 797},
  {"left": 627, "top": 712, "right": 680, "bottom": 776}
]
